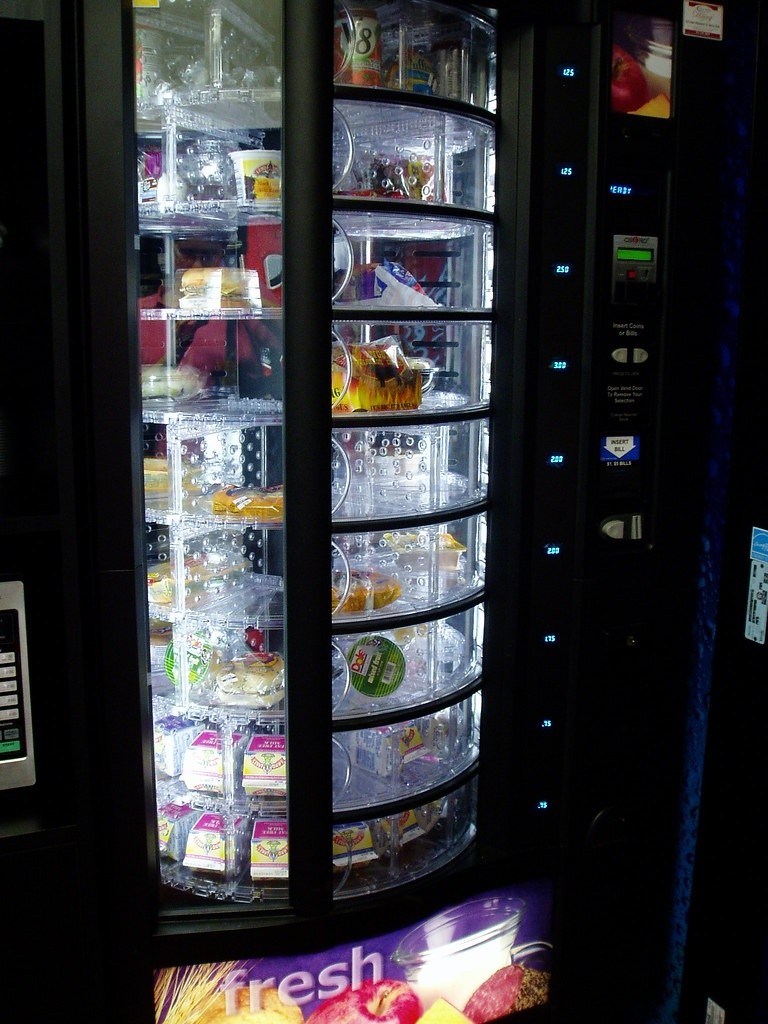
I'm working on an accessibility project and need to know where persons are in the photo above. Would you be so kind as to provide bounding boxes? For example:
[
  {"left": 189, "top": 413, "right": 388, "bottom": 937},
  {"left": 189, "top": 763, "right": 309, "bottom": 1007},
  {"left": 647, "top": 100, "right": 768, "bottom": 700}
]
[{"left": 138, "top": 239, "right": 276, "bottom": 389}]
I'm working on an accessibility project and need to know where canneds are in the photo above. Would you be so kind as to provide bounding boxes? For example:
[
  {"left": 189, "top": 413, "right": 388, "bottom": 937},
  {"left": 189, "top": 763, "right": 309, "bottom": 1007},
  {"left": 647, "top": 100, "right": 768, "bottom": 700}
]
[{"left": 332, "top": 7, "right": 382, "bottom": 87}]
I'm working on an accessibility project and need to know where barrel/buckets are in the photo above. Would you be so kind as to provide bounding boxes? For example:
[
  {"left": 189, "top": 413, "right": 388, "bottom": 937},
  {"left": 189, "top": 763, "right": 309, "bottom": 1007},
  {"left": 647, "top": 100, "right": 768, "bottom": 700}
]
[{"left": 228, "top": 150, "right": 281, "bottom": 201}]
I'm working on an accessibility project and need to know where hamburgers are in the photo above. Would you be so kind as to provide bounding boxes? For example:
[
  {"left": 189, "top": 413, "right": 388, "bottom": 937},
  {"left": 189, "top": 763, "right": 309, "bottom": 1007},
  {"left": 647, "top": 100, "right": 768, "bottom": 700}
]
[{"left": 178, "top": 267, "right": 251, "bottom": 309}]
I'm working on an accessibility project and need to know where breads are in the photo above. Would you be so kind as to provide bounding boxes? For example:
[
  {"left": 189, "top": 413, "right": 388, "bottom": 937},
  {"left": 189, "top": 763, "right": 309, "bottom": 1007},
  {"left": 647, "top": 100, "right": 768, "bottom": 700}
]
[
  {"left": 210, "top": 490, "right": 285, "bottom": 518},
  {"left": 216, "top": 650, "right": 285, "bottom": 708},
  {"left": 327, "top": 349, "right": 422, "bottom": 412}
]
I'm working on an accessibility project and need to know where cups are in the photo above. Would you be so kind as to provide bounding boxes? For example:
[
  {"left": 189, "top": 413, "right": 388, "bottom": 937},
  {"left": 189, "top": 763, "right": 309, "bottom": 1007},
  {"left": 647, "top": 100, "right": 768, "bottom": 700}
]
[{"left": 391, "top": 897, "right": 552, "bottom": 1012}]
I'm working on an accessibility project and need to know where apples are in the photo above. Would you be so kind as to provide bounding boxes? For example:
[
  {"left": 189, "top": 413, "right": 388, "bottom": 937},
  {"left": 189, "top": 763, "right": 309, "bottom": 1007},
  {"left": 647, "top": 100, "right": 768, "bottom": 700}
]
[
  {"left": 304, "top": 979, "right": 423, "bottom": 1024},
  {"left": 611, "top": 45, "right": 670, "bottom": 119}
]
